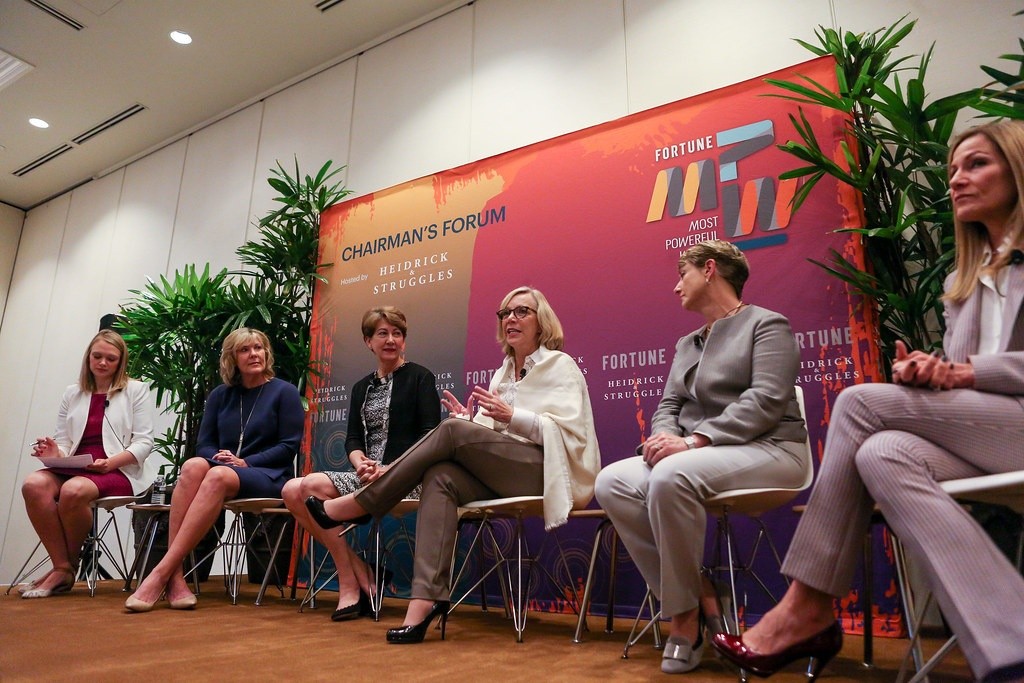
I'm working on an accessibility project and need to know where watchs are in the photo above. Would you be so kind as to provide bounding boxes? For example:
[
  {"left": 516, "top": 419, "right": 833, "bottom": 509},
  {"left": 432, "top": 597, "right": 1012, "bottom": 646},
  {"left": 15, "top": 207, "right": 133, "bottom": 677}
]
[{"left": 685, "top": 437, "right": 696, "bottom": 449}]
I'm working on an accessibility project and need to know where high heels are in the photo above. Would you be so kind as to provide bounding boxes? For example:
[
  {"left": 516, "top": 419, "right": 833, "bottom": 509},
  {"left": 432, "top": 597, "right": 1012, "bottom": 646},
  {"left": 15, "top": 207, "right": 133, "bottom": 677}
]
[
  {"left": 387, "top": 600, "right": 450, "bottom": 643},
  {"left": 711, "top": 620, "right": 842, "bottom": 683},
  {"left": 305, "top": 495, "right": 371, "bottom": 536}
]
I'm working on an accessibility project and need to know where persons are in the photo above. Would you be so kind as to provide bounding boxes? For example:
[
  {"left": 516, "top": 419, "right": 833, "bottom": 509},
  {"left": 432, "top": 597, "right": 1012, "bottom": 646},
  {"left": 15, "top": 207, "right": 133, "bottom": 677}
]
[
  {"left": 17, "top": 328, "right": 155, "bottom": 599},
  {"left": 124, "top": 327, "right": 306, "bottom": 612},
  {"left": 302, "top": 284, "right": 602, "bottom": 648},
  {"left": 709, "top": 120, "right": 1023, "bottom": 683},
  {"left": 280, "top": 305, "right": 441, "bottom": 620},
  {"left": 594, "top": 238, "right": 807, "bottom": 674}
]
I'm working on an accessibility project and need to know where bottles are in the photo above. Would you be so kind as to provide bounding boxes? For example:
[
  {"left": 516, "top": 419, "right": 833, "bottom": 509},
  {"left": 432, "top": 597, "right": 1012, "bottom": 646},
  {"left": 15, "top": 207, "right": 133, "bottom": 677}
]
[{"left": 151, "top": 475, "right": 167, "bottom": 504}]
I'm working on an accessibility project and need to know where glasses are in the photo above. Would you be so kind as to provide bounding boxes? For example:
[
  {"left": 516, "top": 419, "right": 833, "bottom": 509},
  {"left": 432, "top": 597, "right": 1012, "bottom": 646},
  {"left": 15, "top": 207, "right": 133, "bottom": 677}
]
[{"left": 497, "top": 306, "right": 537, "bottom": 321}]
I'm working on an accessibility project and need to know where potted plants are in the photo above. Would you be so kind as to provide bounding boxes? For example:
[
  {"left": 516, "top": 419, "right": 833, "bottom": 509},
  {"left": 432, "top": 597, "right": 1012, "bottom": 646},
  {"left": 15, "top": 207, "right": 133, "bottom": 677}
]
[
  {"left": 758, "top": 8, "right": 986, "bottom": 635},
  {"left": 110, "top": 150, "right": 356, "bottom": 583}
]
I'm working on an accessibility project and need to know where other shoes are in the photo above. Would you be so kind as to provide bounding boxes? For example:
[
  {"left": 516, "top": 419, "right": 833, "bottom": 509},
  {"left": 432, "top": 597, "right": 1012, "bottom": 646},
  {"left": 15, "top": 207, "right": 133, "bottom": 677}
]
[
  {"left": 703, "top": 575, "right": 738, "bottom": 659},
  {"left": 125, "top": 581, "right": 166, "bottom": 612},
  {"left": 22, "top": 567, "right": 75, "bottom": 597},
  {"left": 165, "top": 589, "right": 198, "bottom": 609},
  {"left": 361, "top": 564, "right": 384, "bottom": 607},
  {"left": 18, "top": 571, "right": 54, "bottom": 594},
  {"left": 661, "top": 615, "right": 703, "bottom": 673},
  {"left": 332, "top": 590, "right": 369, "bottom": 619}
]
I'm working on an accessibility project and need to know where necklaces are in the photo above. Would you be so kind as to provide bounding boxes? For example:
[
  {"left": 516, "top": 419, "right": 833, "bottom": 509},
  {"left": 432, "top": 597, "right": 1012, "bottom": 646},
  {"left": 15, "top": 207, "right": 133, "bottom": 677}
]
[{"left": 235, "top": 383, "right": 265, "bottom": 458}]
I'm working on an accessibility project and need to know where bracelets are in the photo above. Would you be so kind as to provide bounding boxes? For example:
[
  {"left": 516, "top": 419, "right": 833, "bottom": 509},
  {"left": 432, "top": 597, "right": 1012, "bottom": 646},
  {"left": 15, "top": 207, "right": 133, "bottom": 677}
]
[{"left": 929, "top": 383, "right": 941, "bottom": 391}]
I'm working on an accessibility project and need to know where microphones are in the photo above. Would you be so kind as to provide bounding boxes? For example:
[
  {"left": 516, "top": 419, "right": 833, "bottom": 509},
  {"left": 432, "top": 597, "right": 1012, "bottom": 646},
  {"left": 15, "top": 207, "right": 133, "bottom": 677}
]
[
  {"left": 1011, "top": 249, "right": 1024, "bottom": 265},
  {"left": 519, "top": 368, "right": 526, "bottom": 377},
  {"left": 105, "top": 400, "right": 109, "bottom": 407},
  {"left": 694, "top": 334, "right": 700, "bottom": 346},
  {"left": 373, "top": 377, "right": 380, "bottom": 387}
]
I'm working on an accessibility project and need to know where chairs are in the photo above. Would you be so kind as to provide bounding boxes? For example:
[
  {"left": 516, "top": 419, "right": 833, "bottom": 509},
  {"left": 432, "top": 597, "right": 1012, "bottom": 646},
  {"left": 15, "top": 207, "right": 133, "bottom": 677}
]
[{"left": 5, "top": 385, "right": 1024, "bottom": 683}]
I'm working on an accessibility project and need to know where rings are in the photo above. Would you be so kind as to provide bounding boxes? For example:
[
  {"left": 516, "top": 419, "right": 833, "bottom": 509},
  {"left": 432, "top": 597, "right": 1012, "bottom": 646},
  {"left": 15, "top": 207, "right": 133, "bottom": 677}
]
[
  {"left": 488, "top": 404, "right": 492, "bottom": 411},
  {"left": 655, "top": 442, "right": 660, "bottom": 450}
]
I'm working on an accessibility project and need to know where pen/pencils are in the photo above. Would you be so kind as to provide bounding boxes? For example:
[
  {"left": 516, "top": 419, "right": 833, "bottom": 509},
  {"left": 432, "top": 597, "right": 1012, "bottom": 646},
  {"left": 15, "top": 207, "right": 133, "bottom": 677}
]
[{"left": 29, "top": 437, "right": 56, "bottom": 447}]
